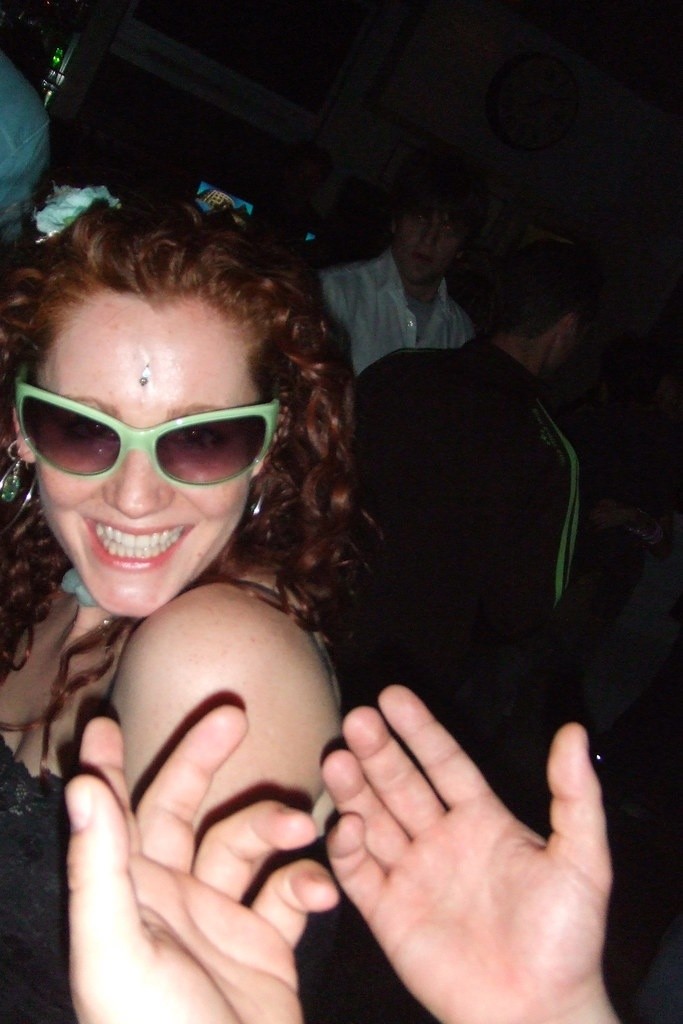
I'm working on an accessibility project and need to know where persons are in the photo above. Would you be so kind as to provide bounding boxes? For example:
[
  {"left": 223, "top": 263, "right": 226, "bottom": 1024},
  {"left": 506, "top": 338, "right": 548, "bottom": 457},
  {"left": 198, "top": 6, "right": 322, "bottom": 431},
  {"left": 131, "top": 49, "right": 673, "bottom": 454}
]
[
  {"left": 65, "top": 681, "right": 621, "bottom": 1024},
  {"left": 0, "top": 191, "right": 361, "bottom": 1024},
  {"left": 0, "top": 21, "right": 682, "bottom": 1024}
]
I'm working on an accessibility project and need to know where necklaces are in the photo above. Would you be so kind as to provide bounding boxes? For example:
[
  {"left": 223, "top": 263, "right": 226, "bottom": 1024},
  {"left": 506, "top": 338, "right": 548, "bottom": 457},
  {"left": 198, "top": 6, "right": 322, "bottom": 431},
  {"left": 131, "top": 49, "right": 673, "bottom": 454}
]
[{"left": 92, "top": 618, "right": 110, "bottom": 634}]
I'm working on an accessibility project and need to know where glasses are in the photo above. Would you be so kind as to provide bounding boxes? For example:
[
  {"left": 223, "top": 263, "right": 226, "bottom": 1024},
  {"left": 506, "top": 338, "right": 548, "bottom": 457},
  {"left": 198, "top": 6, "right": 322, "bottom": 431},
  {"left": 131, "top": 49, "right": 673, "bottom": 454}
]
[{"left": 14, "top": 363, "right": 282, "bottom": 489}]
